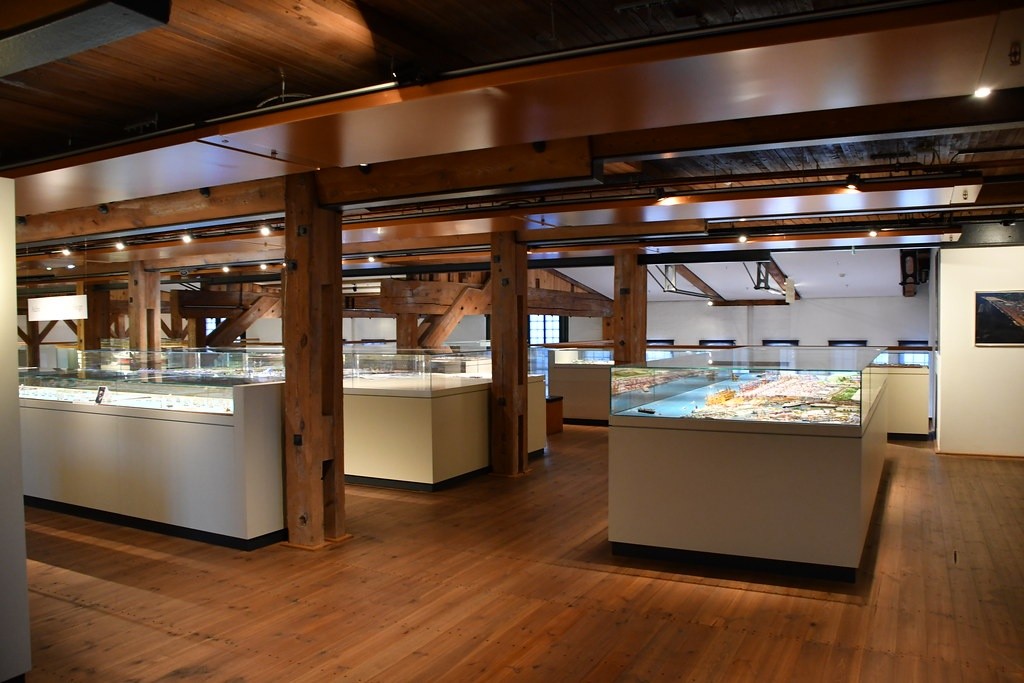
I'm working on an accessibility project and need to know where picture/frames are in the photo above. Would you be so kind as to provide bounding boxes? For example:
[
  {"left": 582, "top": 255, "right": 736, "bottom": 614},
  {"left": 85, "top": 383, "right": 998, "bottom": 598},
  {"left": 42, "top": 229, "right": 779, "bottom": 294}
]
[{"left": 973, "top": 290, "right": 1024, "bottom": 347}]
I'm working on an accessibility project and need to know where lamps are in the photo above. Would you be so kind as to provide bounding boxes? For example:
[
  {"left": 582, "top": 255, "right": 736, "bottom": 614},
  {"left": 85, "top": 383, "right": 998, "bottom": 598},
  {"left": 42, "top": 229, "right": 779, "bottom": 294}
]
[{"left": 845, "top": 174, "right": 859, "bottom": 189}]
